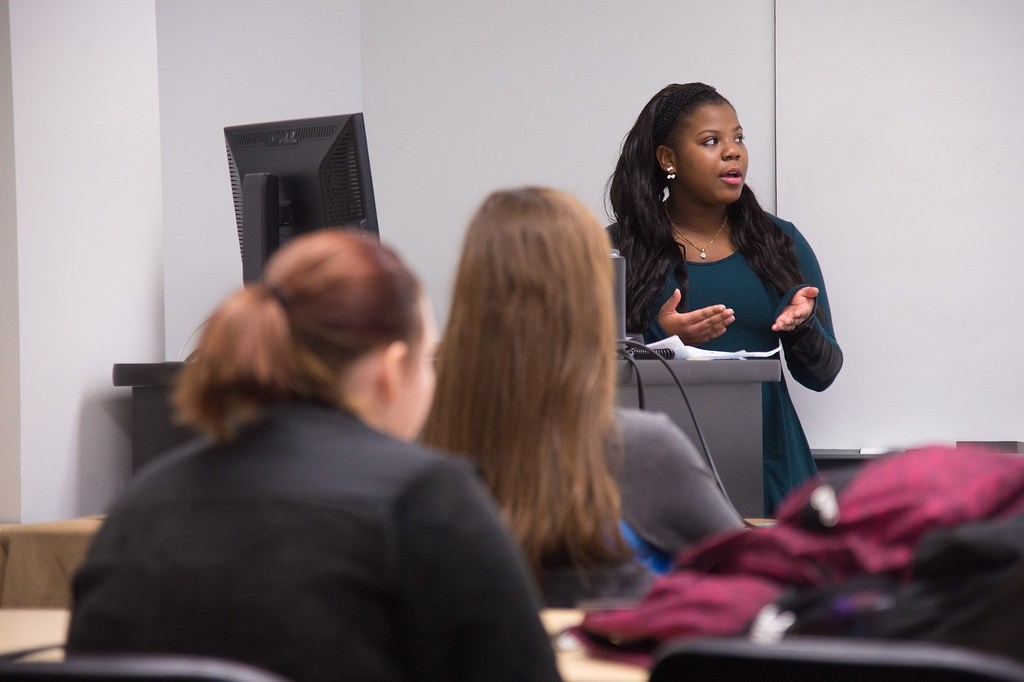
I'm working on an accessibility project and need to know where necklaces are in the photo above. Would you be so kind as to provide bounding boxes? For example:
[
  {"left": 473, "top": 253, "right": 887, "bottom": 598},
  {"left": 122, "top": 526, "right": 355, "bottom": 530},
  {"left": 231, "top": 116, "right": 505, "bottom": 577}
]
[{"left": 663, "top": 216, "right": 729, "bottom": 260}]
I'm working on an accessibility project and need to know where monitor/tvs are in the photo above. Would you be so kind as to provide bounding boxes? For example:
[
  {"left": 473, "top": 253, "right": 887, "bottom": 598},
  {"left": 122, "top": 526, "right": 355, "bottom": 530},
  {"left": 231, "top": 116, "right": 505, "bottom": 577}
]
[{"left": 226, "top": 113, "right": 380, "bottom": 289}]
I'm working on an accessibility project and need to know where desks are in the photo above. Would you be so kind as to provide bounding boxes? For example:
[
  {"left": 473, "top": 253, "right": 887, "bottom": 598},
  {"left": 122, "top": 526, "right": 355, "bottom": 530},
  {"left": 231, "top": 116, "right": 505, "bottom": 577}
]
[{"left": 0, "top": 606, "right": 651, "bottom": 682}]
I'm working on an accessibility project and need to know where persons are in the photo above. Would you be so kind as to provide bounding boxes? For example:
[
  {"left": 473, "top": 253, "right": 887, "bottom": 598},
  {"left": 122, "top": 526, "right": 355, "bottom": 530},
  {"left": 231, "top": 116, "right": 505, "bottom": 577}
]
[
  {"left": 416, "top": 182, "right": 750, "bottom": 606},
  {"left": 66, "top": 223, "right": 564, "bottom": 682},
  {"left": 600, "top": 81, "right": 845, "bottom": 523}
]
